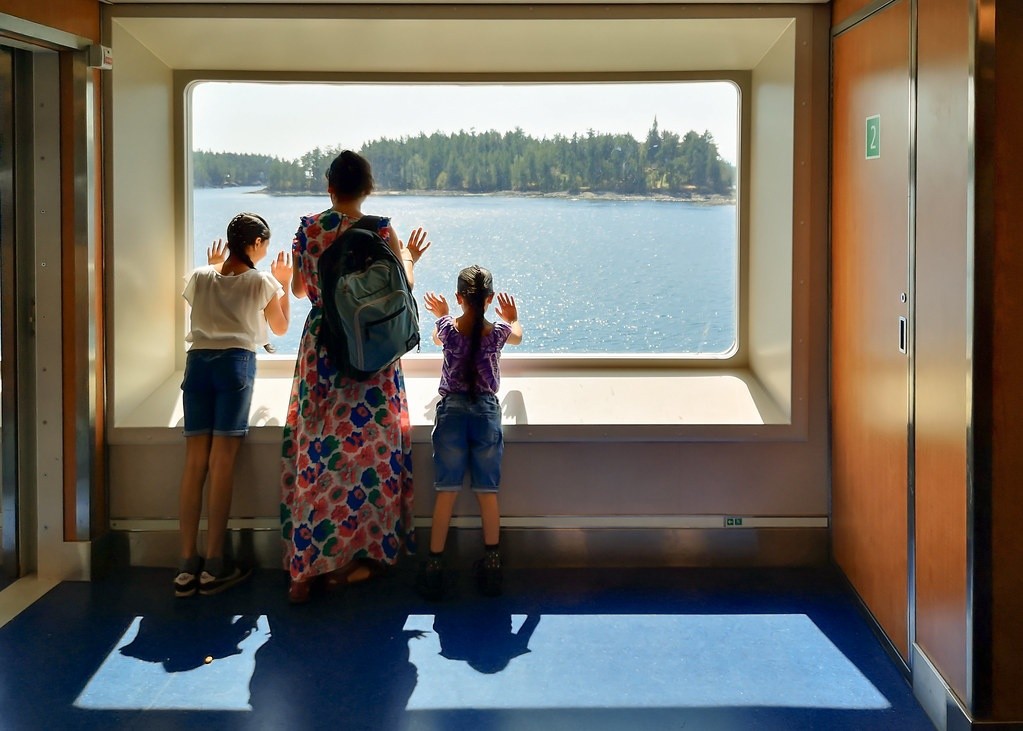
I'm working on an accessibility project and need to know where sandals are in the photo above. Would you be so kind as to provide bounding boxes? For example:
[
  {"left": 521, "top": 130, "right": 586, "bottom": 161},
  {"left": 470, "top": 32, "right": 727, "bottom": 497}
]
[
  {"left": 287, "top": 579, "right": 311, "bottom": 602},
  {"left": 328, "top": 563, "right": 384, "bottom": 589}
]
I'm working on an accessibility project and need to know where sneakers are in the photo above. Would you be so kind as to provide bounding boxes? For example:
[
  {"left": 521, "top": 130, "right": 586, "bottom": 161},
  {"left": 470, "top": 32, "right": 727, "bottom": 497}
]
[
  {"left": 201, "top": 565, "right": 251, "bottom": 595},
  {"left": 175, "top": 557, "right": 206, "bottom": 597}
]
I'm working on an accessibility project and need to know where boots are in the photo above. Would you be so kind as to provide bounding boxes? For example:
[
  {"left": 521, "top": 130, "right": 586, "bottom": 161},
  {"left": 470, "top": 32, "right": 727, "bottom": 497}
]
[
  {"left": 472, "top": 542, "right": 505, "bottom": 596},
  {"left": 416, "top": 552, "right": 448, "bottom": 602}
]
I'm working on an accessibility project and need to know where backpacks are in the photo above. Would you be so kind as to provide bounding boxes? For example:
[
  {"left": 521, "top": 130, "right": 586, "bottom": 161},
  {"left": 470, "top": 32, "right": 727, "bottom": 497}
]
[{"left": 316, "top": 216, "right": 421, "bottom": 380}]
